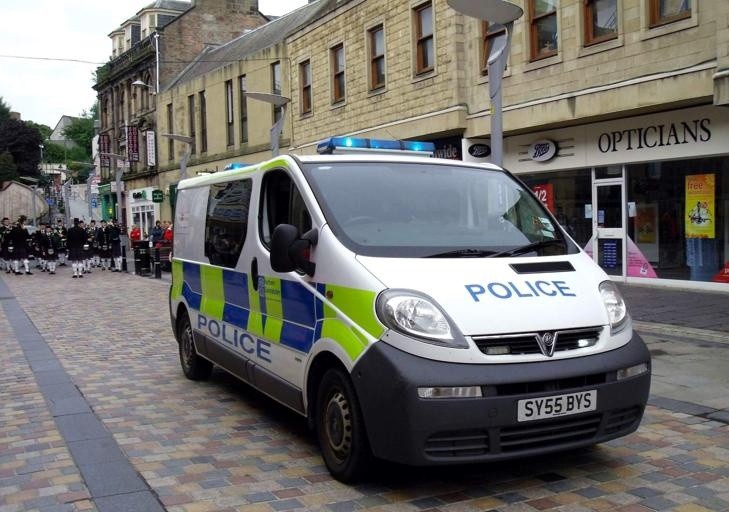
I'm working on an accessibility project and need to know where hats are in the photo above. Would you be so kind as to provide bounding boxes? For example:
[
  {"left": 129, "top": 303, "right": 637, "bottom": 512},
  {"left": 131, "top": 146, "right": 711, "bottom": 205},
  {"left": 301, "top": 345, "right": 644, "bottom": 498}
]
[
  {"left": 46, "top": 225, "right": 50, "bottom": 228},
  {"left": 74, "top": 218, "right": 83, "bottom": 223},
  {"left": 58, "top": 220, "right": 61, "bottom": 222}
]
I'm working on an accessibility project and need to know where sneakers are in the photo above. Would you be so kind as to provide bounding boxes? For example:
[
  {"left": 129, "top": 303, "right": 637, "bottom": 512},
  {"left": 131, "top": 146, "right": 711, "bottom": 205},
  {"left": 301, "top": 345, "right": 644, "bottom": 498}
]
[
  {"left": 37, "top": 263, "right": 67, "bottom": 274},
  {"left": 5, "top": 265, "right": 33, "bottom": 274},
  {"left": 73, "top": 264, "right": 122, "bottom": 278}
]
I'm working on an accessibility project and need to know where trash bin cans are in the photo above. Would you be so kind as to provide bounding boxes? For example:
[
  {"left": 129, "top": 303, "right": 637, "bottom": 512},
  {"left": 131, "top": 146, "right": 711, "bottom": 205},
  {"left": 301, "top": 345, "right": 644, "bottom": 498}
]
[{"left": 133, "top": 240, "right": 150, "bottom": 274}]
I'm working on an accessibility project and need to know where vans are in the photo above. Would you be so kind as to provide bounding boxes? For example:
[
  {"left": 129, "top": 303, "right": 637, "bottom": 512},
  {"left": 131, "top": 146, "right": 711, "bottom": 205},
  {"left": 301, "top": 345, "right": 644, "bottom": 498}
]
[{"left": 167, "top": 135, "right": 653, "bottom": 483}]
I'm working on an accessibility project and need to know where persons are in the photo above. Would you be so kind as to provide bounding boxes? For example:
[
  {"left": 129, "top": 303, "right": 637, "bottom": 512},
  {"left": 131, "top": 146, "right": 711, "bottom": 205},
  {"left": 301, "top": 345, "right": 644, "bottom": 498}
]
[
  {"left": 131, "top": 224, "right": 141, "bottom": 240},
  {"left": 0, "top": 214, "right": 128, "bottom": 279},
  {"left": 153, "top": 220, "right": 174, "bottom": 247}
]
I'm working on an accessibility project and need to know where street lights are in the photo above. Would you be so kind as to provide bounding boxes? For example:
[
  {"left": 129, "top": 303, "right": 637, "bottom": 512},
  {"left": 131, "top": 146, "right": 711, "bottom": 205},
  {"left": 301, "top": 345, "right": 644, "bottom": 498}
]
[
  {"left": 100, "top": 153, "right": 129, "bottom": 223},
  {"left": 447, "top": 0, "right": 523, "bottom": 167},
  {"left": 242, "top": 91, "right": 292, "bottom": 158},
  {"left": 162, "top": 133, "right": 193, "bottom": 180}
]
